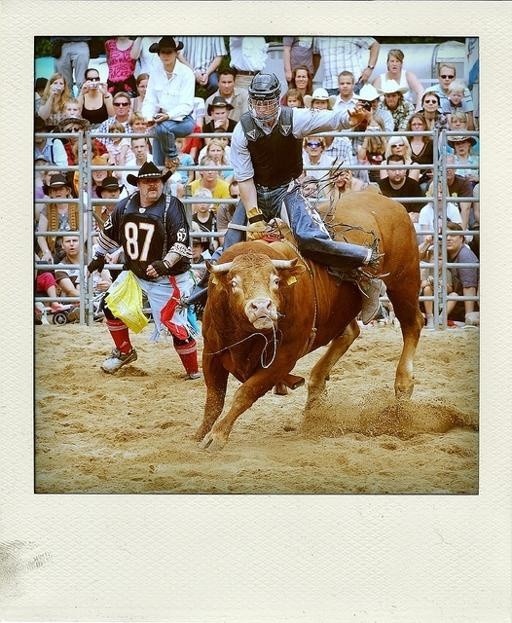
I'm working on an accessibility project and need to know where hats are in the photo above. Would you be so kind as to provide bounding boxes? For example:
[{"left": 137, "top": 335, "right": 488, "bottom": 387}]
[
  {"left": 149, "top": 37, "right": 184, "bottom": 53},
  {"left": 207, "top": 96, "right": 233, "bottom": 116},
  {"left": 354, "top": 79, "right": 409, "bottom": 101},
  {"left": 447, "top": 135, "right": 477, "bottom": 149},
  {"left": 42, "top": 161, "right": 173, "bottom": 198},
  {"left": 59, "top": 113, "right": 90, "bottom": 128},
  {"left": 303, "top": 88, "right": 336, "bottom": 109}
]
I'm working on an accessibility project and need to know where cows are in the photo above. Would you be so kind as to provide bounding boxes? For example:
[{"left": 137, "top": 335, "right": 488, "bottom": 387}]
[{"left": 193, "top": 188, "right": 427, "bottom": 455}]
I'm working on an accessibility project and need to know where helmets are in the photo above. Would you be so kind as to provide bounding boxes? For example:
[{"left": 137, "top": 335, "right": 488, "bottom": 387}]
[{"left": 247, "top": 73, "right": 281, "bottom": 122}]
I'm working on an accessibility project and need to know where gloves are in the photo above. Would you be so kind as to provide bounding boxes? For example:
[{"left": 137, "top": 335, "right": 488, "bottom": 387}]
[{"left": 246, "top": 208, "right": 266, "bottom": 240}]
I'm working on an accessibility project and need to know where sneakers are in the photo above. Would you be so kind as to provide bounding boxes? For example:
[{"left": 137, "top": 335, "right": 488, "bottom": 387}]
[
  {"left": 50, "top": 302, "right": 70, "bottom": 313},
  {"left": 101, "top": 349, "right": 137, "bottom": 373},
  {"left": 361, "top": 278, "right": 387, "bottom": 325}
]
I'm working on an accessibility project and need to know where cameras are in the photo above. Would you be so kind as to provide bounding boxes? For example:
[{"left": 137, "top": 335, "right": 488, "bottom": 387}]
[
  {"left": 56, "top": 88, "right": 62, "bottom": 93},
  {"left": 89, "top": 83, "right": 98, "bottom": 89},
  {"left": 363, "top": 101, "right": 371, "bottom": 111},
  {"left": 432, "top": 235, "right": 442, "bottom": 242},
  {"left": 154, "top": 112, "right": 160, "bottom": 119}
]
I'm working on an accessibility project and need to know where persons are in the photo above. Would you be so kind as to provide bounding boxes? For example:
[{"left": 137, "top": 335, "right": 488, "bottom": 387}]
[
  {"left": 34, "top": 36, "right": 479, "bottom": 324},
  {"left": 85, "top": 161, "right": 205, "bottom": 380}
]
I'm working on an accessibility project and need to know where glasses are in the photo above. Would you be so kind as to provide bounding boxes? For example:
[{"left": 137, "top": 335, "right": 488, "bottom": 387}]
[
  {"left": 113, "top": 103, "right": 129, "bottom": 106},
  {"left": 306, "top": 142, "right": 321, "bottom": 147},
  {"left": 440, "top": 75, "right": 454, "bottom": 78},
  {"left": 67, "top": 128, "right": 78, "bottom": 132},
  {"left": 87, "top": 77, "right": 100, "bottom": 80},
  {"left": 425, "top": 100, "right": 436, "bottom": 103},
  {"left": 391, "top": 144, "right": 404, "bottom": 147}
]
